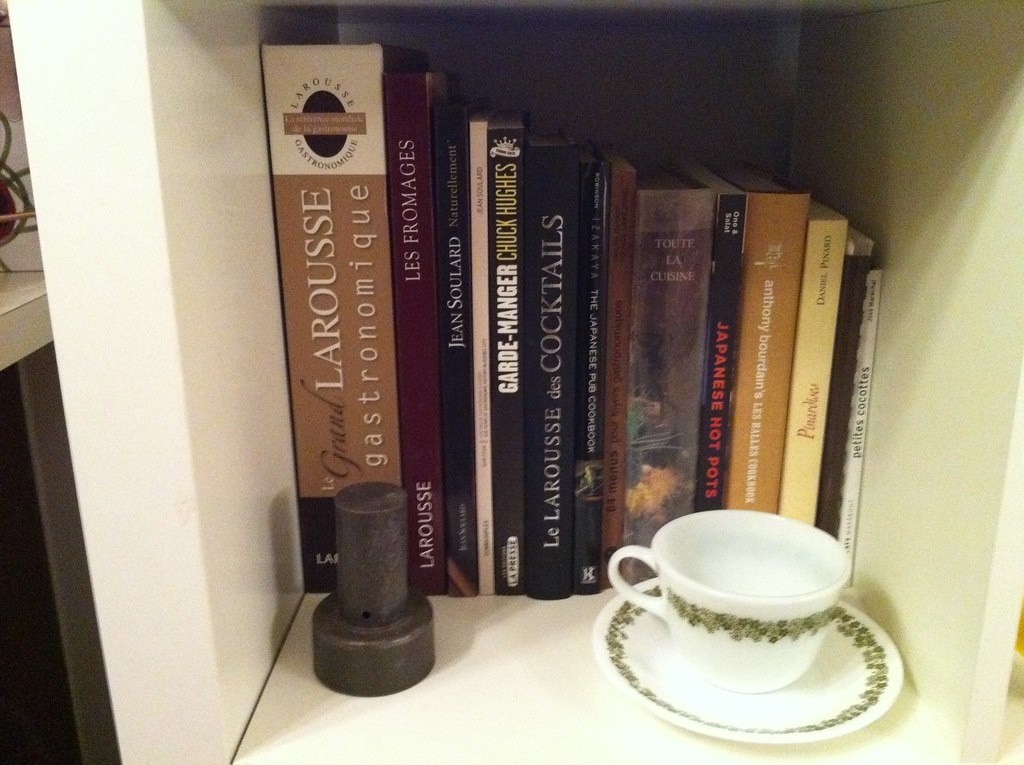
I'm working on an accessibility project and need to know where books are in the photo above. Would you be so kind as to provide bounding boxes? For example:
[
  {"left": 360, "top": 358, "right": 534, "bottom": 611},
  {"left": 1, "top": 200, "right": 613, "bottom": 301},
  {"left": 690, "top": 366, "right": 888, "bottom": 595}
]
[{"left": 262, "top": 41, "right": 884, "bottom": 602}]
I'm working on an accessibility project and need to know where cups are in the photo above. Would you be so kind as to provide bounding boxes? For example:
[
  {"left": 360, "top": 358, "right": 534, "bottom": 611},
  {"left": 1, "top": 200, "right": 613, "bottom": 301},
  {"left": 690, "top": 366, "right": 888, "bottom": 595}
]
[{"left": 608, "top": 510, "right": 851, "bottom": 693}]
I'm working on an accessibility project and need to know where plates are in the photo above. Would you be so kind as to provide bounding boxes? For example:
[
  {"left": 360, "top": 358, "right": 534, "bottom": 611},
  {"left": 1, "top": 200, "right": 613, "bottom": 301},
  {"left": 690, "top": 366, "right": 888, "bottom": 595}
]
[{"left": 592, "top": 578, "right": 904, "bottom": 743}]
